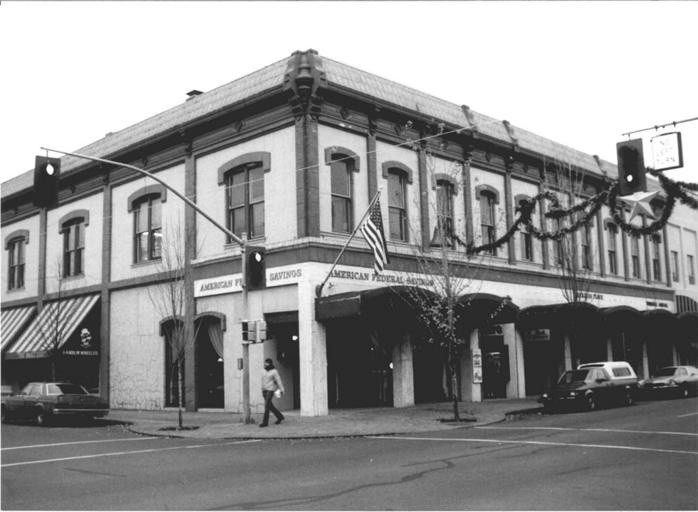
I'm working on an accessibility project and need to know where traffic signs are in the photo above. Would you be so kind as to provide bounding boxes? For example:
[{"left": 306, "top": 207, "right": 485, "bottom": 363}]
[{"left": 653, "top": 134, "right": 681, "bottom": 169}]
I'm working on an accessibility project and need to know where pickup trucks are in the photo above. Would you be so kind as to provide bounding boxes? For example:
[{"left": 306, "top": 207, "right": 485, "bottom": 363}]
[{"left": 538, "top": 360, "right": 639, "bottom": 410}]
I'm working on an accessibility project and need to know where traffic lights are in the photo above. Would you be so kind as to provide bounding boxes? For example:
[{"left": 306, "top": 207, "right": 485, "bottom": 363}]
[
  {"left": 240, "top": 321, "right": 256, "bottom": 344},
  {"left": 617, "top": 138, "right": 647, "bottom": 195},
  {"left": 261, "top": 320, "right": 273, "bottom": 341},
  {"left": 244, "top": 246, "right": 268, "bottom": 287},
  {"left": 34, "top": 156, "right": 60, "bottom": 208}
]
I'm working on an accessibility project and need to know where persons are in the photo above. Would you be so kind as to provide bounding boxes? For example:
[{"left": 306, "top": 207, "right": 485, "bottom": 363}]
[{"left": 259, "top": 358, "right": 285, "bottom": 427}]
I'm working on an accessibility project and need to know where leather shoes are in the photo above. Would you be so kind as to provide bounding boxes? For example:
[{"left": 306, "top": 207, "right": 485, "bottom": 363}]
[
  {"left": 275, "top": 416, "right": 284, "bottom": 424},
  {"left": 259, "top": 422, "right": 268, "bottom": 427}
]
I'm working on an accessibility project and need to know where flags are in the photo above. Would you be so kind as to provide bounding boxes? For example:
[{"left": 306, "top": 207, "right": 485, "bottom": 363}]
[{"left": 359, "top": 197, "right": 390, "bottom": 275}]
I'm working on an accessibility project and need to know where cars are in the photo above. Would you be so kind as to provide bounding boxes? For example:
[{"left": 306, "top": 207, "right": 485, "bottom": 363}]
[
  {"left": 1, "top": 382, "right": 110, "bottom": 426},
  {"left": 644, "top": 365, "right": 698, "bottom": 398}
]
